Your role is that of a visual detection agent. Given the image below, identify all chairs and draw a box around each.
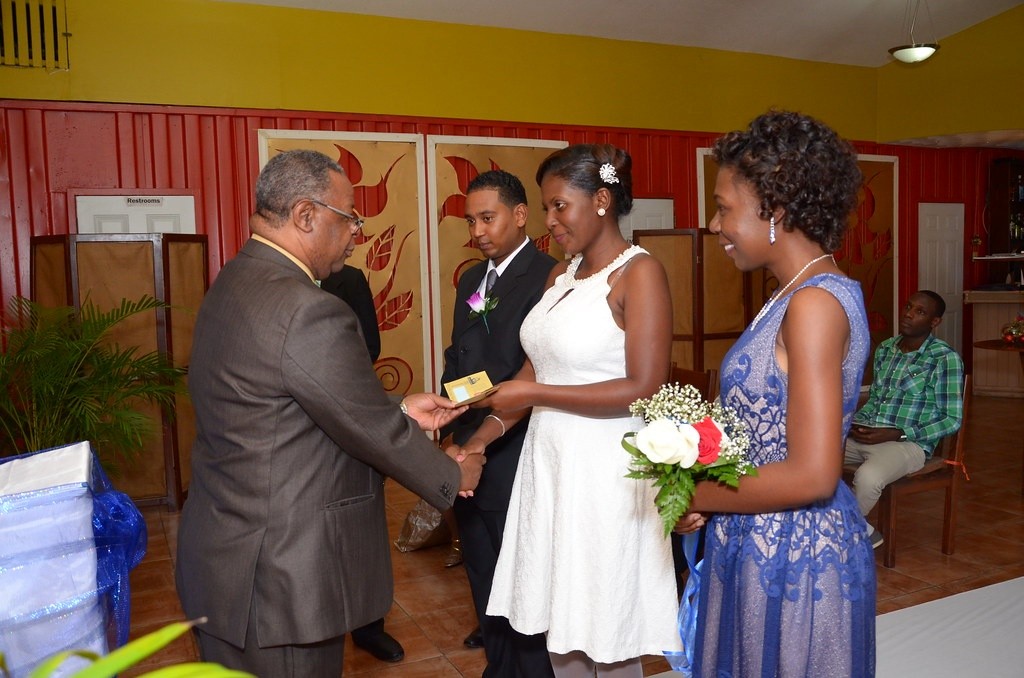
[667,365,718,403]
[840,374,972,569]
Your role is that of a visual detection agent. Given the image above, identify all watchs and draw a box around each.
[894,429,907,443]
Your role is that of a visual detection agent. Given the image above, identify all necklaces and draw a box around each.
[563,242,639,288]
[750,253,834,332]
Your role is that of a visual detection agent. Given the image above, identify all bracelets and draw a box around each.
[400,398,407,415]
[483,415,506,438]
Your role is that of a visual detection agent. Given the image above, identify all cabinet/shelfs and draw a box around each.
[1006,161,1024,289]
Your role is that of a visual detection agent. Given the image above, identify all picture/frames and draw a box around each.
[67,186,203,235]
[426,135,569,440]
[258,129,435,442]
[695,146,899,392]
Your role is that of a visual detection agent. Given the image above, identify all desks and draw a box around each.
[972,340,1024,371]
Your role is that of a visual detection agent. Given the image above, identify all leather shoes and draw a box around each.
[359,631,404,662]
[463,627,484,648]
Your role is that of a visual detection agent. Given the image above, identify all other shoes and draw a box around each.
[443,540,462,566]
[868,528,884,549]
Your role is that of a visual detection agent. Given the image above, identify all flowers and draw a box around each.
[621,381,762,541]
[466,292,500,334]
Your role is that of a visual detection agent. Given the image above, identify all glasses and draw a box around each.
[311,199,364,236]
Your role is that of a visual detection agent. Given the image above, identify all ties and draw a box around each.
[485,269,499,297]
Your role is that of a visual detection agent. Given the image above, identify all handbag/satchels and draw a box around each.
[394,500,450,553]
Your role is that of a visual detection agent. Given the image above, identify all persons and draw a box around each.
[176,149,487,678]
[839,290,966,553]
[640,111,877,678]
[451,145,688,678]
[316,169,557,678]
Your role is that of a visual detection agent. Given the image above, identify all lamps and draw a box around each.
[888,0,941,65]
